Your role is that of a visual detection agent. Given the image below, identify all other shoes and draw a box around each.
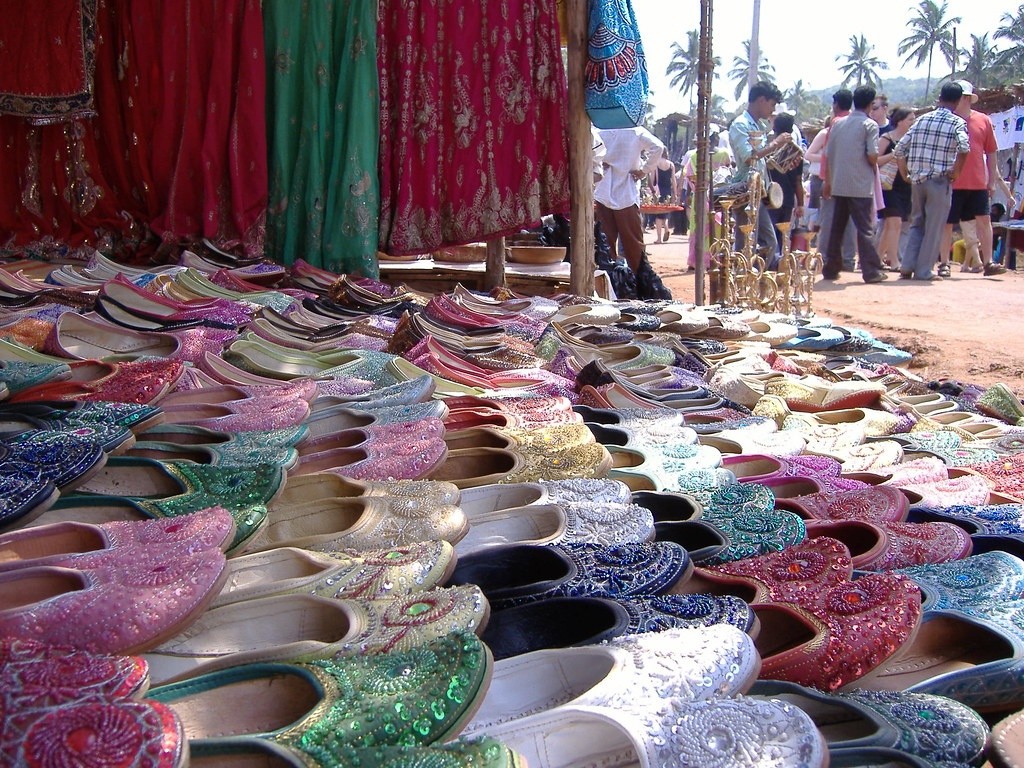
[3,232,1024,768]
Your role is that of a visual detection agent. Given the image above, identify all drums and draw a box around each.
[764,182,784,209]
[768,138,803,176]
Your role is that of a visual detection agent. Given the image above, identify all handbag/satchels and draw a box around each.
[584,1,649,130]
[645,185,659,198]
[879,156,898,190]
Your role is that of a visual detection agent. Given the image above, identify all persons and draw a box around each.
[594,126,663,276]
[640,80,1024,283]
[591,125,607,183]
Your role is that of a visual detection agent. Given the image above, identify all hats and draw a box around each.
[772,103,796,116]
[939,80,978,103]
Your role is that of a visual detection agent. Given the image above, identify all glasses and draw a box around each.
[873,105,881,110]
